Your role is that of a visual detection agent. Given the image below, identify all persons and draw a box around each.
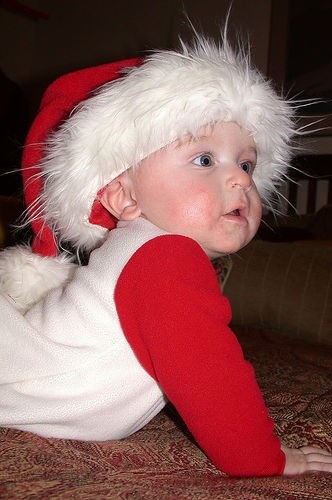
[0,36,332,475]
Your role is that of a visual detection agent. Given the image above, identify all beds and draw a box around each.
[0,155,332,500]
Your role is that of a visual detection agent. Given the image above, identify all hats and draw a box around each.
[0,48,292,310]
